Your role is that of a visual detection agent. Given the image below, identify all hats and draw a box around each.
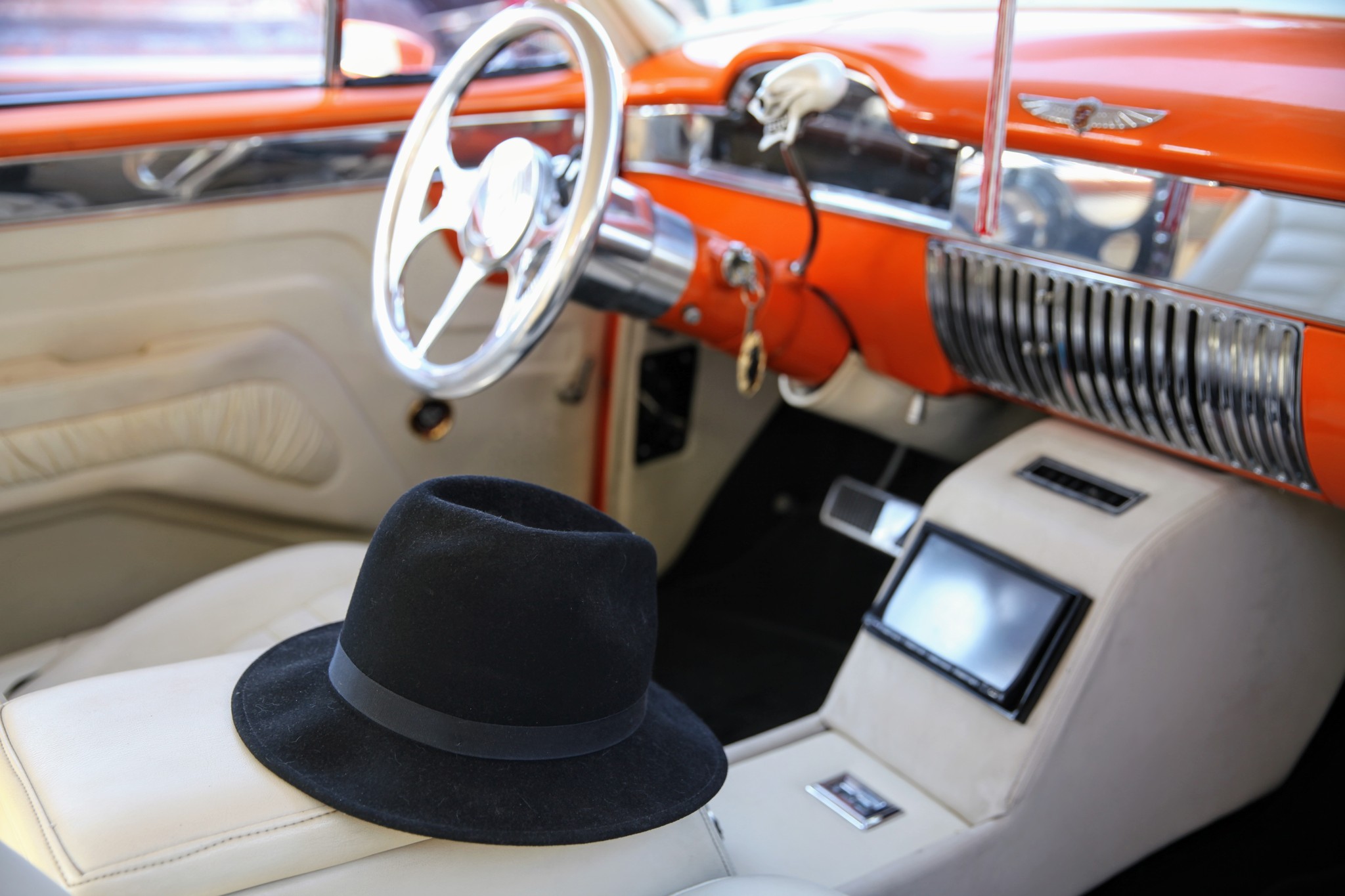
[229,475,729,847]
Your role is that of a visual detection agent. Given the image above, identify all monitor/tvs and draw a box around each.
[860,523,1074,704]
